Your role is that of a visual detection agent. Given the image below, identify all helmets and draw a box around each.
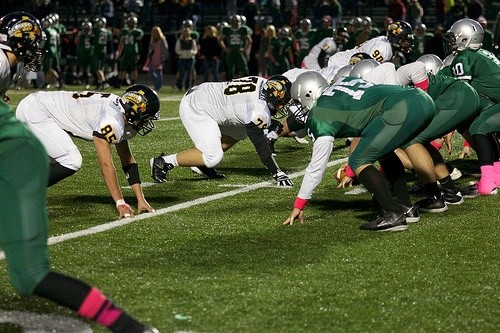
[349,60,379,79]
[40,12,373,38]
[0,11,43,53]
[117,83,161,126]
[387,20,415,46]
[416,53,443,77]
[332,64,354,83]
[291,71,328,112]
[263,75,292,107]
[447,17,484,51]
[442,53,456,67]
[332,26,349,44]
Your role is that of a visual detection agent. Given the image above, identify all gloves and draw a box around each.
[268,118,283,135]
[272,170,294,187]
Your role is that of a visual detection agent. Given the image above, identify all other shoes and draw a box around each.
[293,136,308,144]
[359,205,420,233]
[440,187,465,205]
[262,128,279,156]
[462,179,500,199]
[414,195,449,213]
[437,168,462,184]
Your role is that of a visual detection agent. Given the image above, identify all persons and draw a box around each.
[150,74,294,187]
[16,84,160,220]
[0,0,500,92]
[0,12,48,104]
[283,18,500,233]
[0,100,159,333]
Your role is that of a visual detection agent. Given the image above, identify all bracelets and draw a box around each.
[116,199,125,206]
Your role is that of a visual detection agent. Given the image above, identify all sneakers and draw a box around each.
[191,164,225,179]
[150,152,174,183]
